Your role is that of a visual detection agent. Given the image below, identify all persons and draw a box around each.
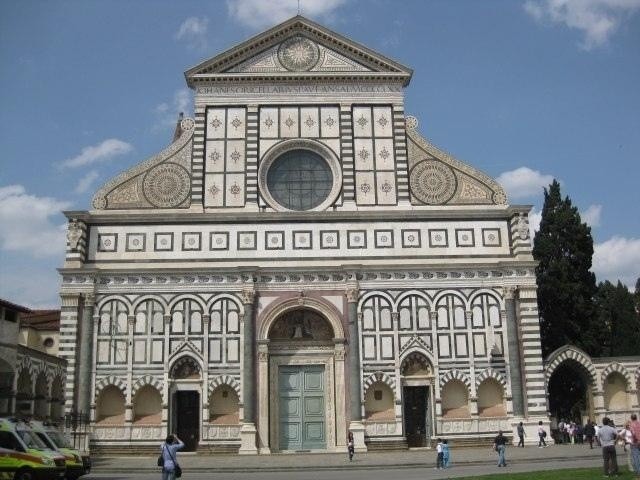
[550,417,583,445]
[346,432,354,462]
[160,433,185,480]
[584,414,640,478]
[494,431,508,467]
[517,422,527,448]
[434,438,449,470]
[537,421,547,446]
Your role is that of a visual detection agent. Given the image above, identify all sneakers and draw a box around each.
[498,464,506,467]
[601,474,618,477]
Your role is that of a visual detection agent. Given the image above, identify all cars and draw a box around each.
[0,417,91,480]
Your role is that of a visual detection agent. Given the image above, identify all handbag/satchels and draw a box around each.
[176,464,182,478]
[158,456,164,466]
